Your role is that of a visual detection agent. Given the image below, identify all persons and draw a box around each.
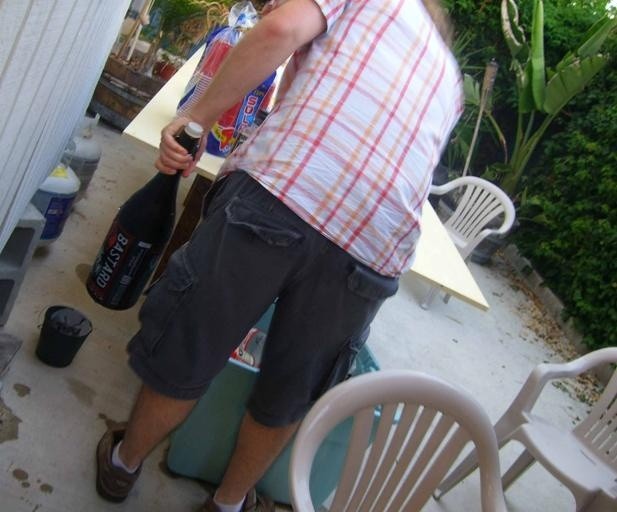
[94,1,466,512]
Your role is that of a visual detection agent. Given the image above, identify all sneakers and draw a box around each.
[94,420,144,504]
[201,488,275,512]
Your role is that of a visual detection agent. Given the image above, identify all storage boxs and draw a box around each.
[164,303,402,512]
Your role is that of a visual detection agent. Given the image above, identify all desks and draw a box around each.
[120,40,492,295]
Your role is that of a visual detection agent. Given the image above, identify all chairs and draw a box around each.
[421,175,517,310]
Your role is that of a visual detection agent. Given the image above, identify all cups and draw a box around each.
[180,38,232,120]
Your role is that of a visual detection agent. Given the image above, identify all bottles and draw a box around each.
[82,121,205,314]
[228,109,270,160]
[207,66,277,157]
